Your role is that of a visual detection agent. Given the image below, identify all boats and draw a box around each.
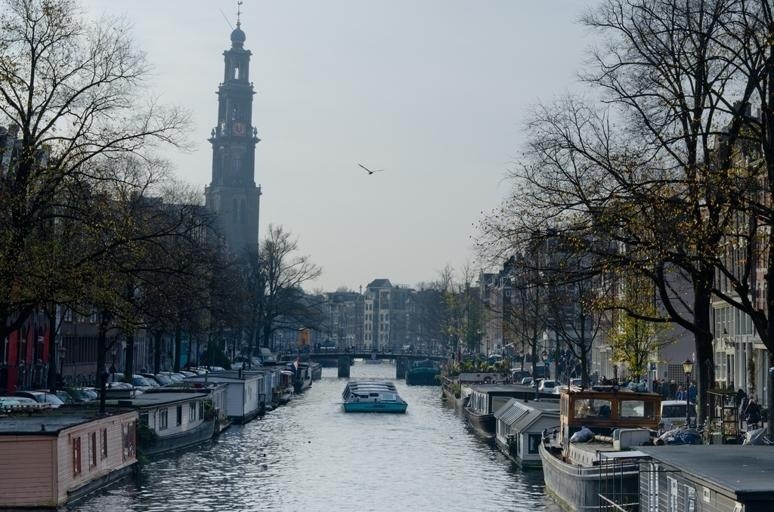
[341,381,408,413]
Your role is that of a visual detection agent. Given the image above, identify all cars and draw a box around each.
[0,345,278,413]
[510,365,698,432]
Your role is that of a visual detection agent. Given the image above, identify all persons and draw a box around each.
[601,379,762,429]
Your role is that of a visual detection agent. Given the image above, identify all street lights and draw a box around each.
[681,357,695,428]
[542,352,548,380]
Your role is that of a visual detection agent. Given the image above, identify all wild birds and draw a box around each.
[358,162,384,175]
